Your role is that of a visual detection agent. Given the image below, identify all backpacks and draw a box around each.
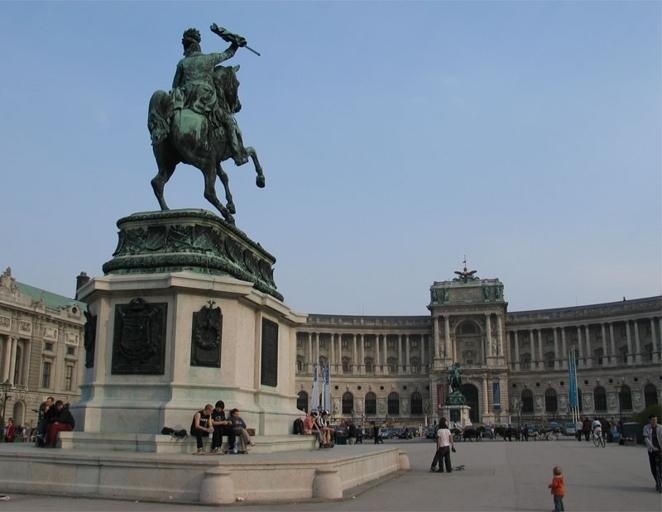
[293,416,305,435]
[161,426,188,441]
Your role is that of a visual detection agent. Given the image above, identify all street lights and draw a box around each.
[2,392,13,420]
[613,381,623,432]
[512,401,524,440]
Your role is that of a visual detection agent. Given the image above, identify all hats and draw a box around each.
[215,400,225,409]
[322,411,328,416]
[311,411,318,418]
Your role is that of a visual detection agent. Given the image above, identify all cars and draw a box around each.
[379,422,576,442]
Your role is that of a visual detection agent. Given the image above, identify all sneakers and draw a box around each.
[319,442,334,448]
[198,441,255,455]
[430,468,453,472]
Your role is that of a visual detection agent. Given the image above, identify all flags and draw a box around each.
[567,349,579,410]
[311,365,331,414]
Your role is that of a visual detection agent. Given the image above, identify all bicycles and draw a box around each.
[592,432,606,448]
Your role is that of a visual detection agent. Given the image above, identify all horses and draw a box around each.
[145,63,266,227]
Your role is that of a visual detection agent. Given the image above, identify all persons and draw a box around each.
[575,415,624,447]
[641,413,662,492]
[211,400,237,454]
[548,466,565,511]
[448,361,465,395]
[1,395,76,448]
[190,403,216,455]
[303,410,564,472]
[170,22,262,166]
[226,407,256,453]
